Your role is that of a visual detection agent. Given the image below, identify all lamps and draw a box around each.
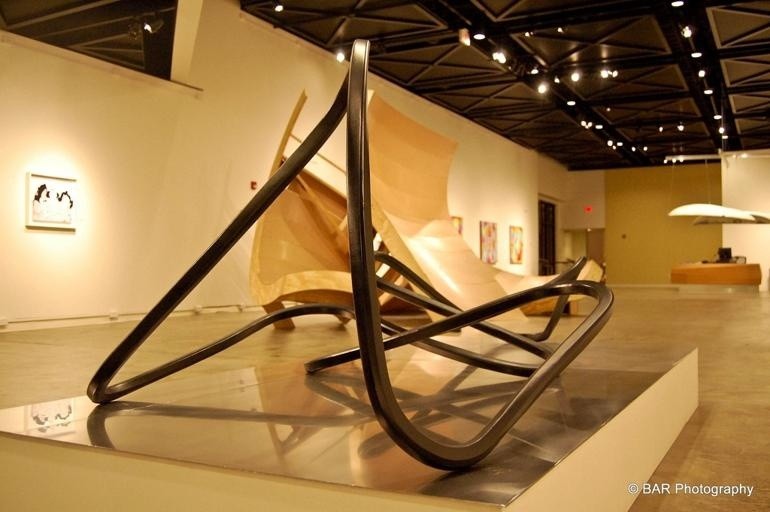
[264,0,740,163]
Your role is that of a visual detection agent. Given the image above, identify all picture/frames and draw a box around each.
[21,171,80,233]
